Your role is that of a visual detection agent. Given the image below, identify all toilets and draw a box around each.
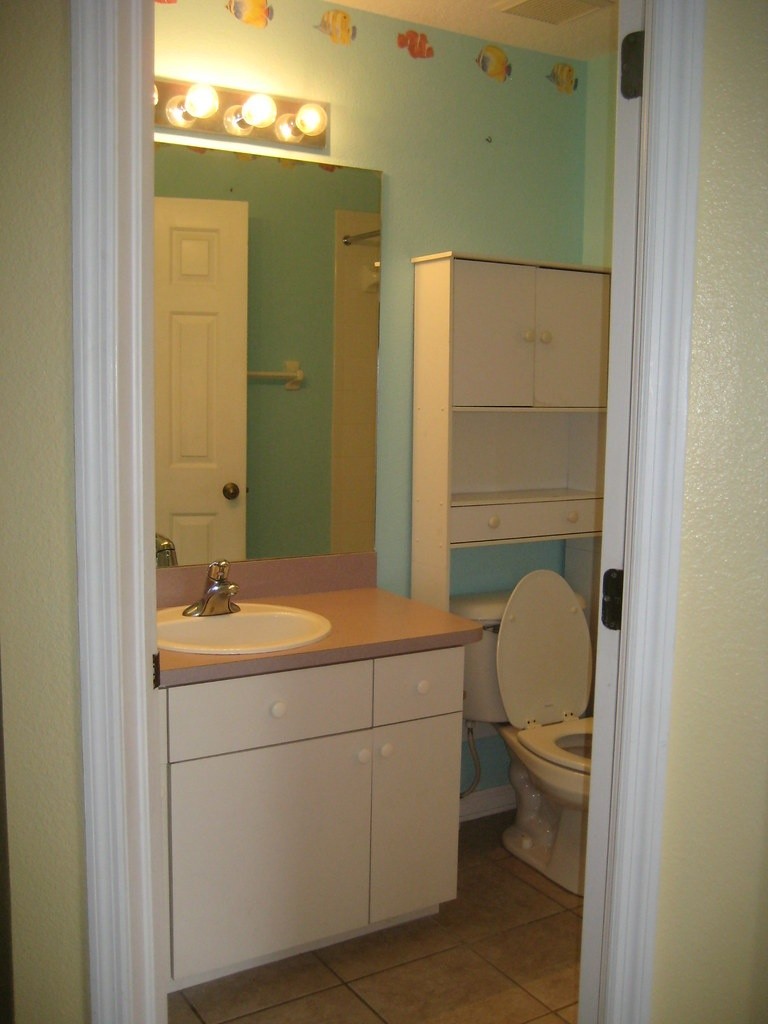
[451,568,595,894]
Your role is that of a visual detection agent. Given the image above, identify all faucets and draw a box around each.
[184,561,240,617]
[157,534,179,567]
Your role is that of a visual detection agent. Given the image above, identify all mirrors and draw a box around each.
[155,139,381,566]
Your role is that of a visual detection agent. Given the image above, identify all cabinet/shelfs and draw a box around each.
[411,252,611,796]
[162,647,466,994]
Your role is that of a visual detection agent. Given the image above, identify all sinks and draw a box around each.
[155,601,334,655]
